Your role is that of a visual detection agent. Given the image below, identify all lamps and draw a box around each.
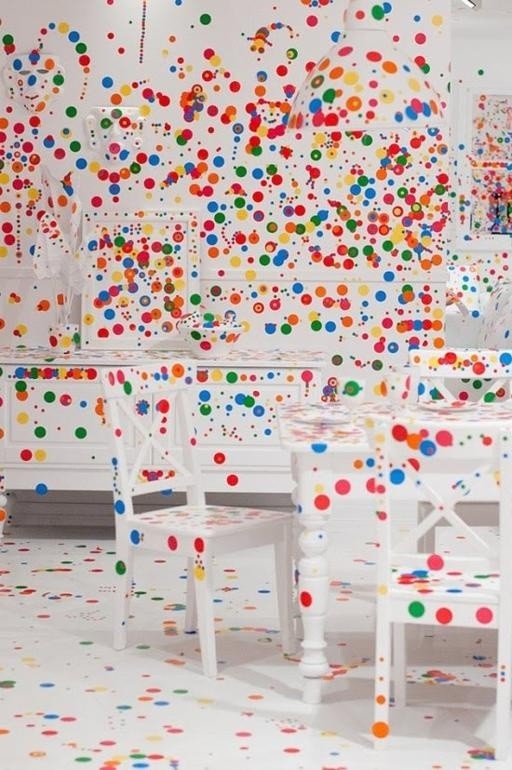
[287,0,448,136]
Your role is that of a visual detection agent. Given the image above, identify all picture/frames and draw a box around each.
[452,73,511,253]
[81,209,202,352]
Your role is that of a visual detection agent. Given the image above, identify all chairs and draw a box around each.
[101,358,297,677]
[365,415,511,762]
[408,348,512,555]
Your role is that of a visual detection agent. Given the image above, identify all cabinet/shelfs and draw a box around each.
[0,345,327,538]
